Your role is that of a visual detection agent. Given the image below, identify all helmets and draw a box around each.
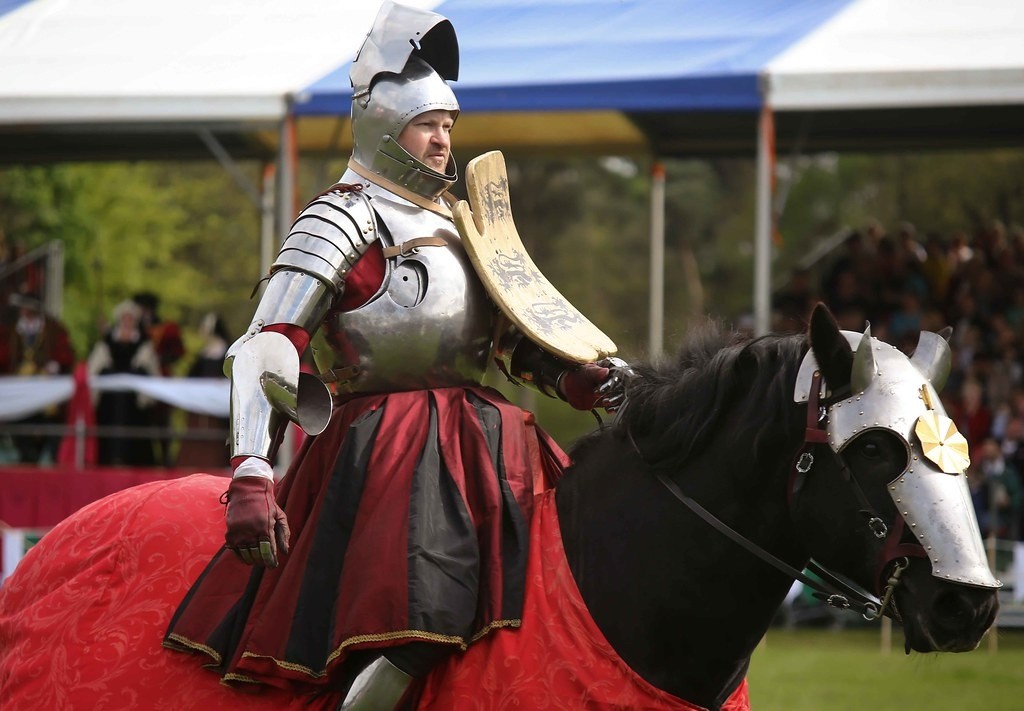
[351,0,461,201]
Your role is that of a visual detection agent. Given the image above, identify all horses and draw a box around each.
[0,299,1001,711]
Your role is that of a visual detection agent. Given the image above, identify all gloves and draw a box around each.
[220,476,291,567]
[563,356,634,415]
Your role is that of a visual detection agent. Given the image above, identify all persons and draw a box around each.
[753,215,1024,567]
[163,4,639,711]
[0,274,235,469]
